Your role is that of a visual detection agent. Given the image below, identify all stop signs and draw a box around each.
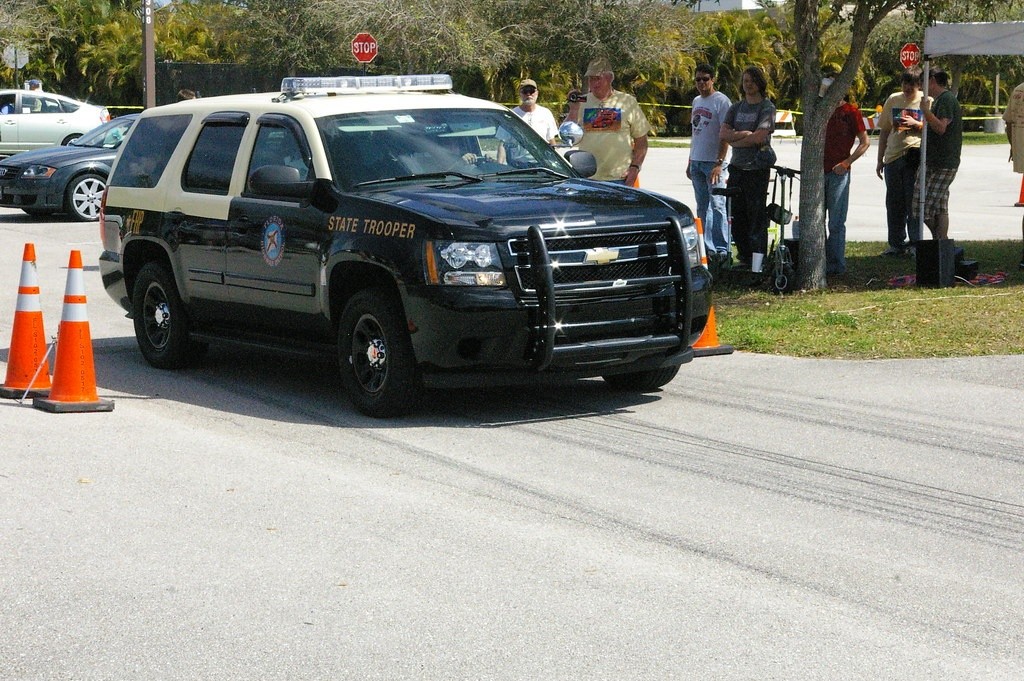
[900,43,921,69]
[351,34,378,63]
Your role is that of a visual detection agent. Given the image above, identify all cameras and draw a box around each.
[570,92,588,103]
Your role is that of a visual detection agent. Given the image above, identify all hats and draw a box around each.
[519,79,537,90]
[584,58,611,76]
[29,80,39,86]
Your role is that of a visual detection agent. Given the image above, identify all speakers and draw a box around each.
[916,238,956,290]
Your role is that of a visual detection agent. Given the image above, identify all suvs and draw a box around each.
[98,73,718,420]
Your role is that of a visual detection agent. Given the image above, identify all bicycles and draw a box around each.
[707,165,803,292]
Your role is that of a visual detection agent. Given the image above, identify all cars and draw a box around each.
[0,113,142,222]
[0,88,111,158]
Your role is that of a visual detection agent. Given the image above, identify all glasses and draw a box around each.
[521,88,536,94]
[695,77,711,82]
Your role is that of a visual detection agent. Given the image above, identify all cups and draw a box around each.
[752,253,763,272]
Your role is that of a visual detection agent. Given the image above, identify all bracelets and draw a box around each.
[840,162,849,169]
[717,159,723,163]
[630,165,639,169]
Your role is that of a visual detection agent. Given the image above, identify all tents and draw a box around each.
[919,17,1024,238]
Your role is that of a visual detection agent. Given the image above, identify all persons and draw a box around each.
[1002,82,1024,266]
[824,95,869,273]
[0,95,15,115]
[563,57,651,187]
[177,89,197,103]
[722,67,775,268]
[495,79,557,166]
[876,66,934,256]
[433,131,478,172]
[912,70,962,240]
[685,64,733,253]
[30,80,43,113]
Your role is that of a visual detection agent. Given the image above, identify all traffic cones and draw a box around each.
[1014,174,1024,208]
[686,215,736,356]
[0,242,53,400]
[33,249,117,413]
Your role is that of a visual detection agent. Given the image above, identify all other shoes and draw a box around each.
[883,247,901,255]
